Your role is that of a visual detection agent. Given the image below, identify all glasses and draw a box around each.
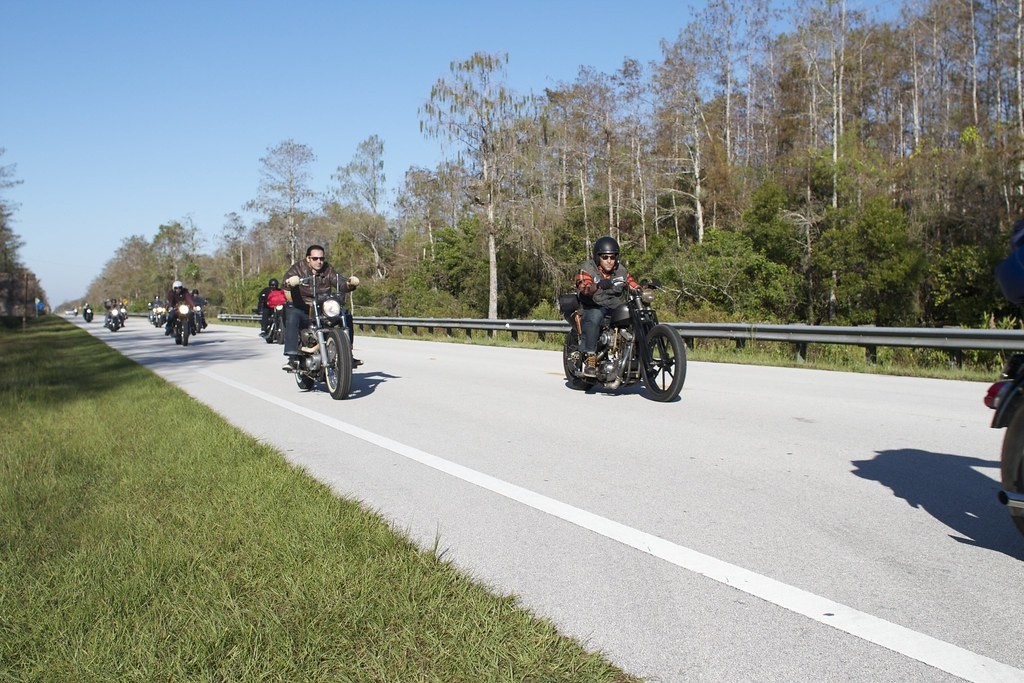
[174,287,182,289]
[270,284,277,287]
[598,254,616,260]
[310,256,326,261]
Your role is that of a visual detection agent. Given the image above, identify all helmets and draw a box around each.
[592,237,621,269]
[269,278,279,289]
[192,289,199,295]
[172,280,184,292]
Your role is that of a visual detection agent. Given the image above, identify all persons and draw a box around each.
[83,303,93,320]
[576,237,643,377]
[259,279,281,335]
[281,245,364,371]
[104,299,126,327]
[149,281,208,335]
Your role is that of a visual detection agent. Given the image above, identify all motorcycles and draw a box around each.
[166,300,196,347]
[289,273,363,399]
[84,308,94,323]
[558,278,688,404]
[105,306,128,333]
[148,303,167,328]
[251,290,293,345]
[984,353,1023,537]
[192,300,209,334]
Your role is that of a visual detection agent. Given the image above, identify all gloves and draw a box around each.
[346,276,361,289]
[285,274,300,286]
[597,278,614,290]
[641,277,662,289]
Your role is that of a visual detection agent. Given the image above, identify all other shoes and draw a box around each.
[352,357,364,368]
[258,332,266,337]
[165,329,171,336]
[283,357,297,372]
[190,330,197,335]
[583,353,597,378]
